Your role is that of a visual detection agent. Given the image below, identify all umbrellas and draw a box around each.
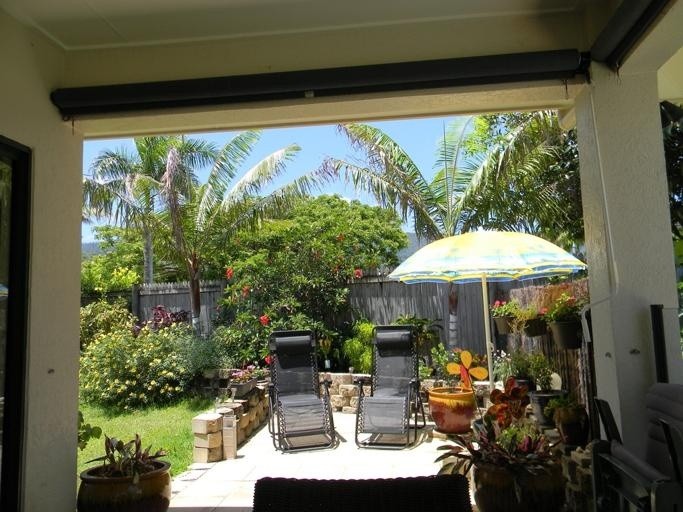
[387,231,588,395]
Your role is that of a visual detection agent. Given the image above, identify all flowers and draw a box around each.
[435,418,556,478]
[231,365,255,384]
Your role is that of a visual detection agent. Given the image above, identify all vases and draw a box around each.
[467,461,568,512]
[229,376,258,397]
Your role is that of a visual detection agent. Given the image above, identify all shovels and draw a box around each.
[592,440,683,512]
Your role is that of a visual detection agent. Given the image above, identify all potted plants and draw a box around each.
[491,293,589,445]
[77,433,172,512]
[428,342,477,434]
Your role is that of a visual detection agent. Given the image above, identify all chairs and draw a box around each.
[252,473,473,511]
[268,330,336,453]
[355,325,426,449]
